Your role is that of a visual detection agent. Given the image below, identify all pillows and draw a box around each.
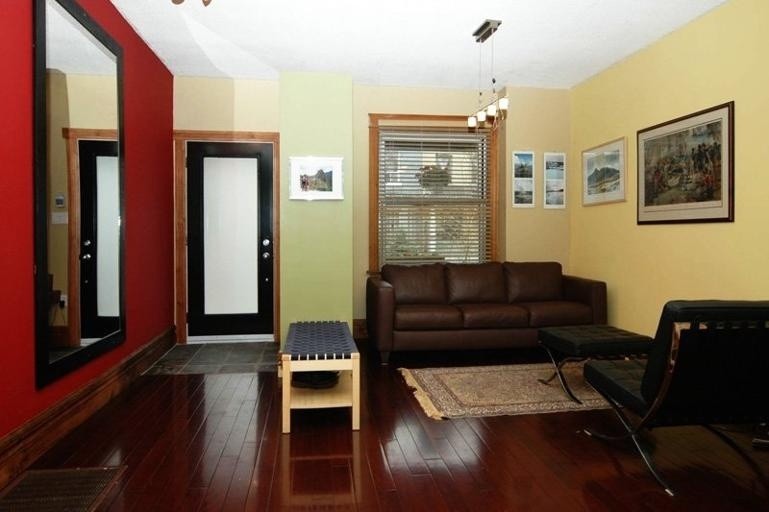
[382,262,447,305]
[445,262,506,305]
[502,261,561,305]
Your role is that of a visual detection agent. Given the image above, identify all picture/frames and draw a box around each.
[512,151,534,209]
[544,153,566,209]
[581,137,628,207]
[638,101,735,225]
[288,155,346,201]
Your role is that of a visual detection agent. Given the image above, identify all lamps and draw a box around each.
[467,19,509,132]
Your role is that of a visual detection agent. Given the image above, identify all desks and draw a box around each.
[278,321,361,434]
[281,434,363,506]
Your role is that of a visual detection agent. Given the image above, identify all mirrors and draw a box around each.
[34,0,127,391]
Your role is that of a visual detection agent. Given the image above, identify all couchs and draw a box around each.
[366,262,607,367]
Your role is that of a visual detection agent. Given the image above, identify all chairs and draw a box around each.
[583,300,768,496]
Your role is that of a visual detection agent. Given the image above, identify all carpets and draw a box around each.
[0,465,128,511]
[397,361,624,421]
